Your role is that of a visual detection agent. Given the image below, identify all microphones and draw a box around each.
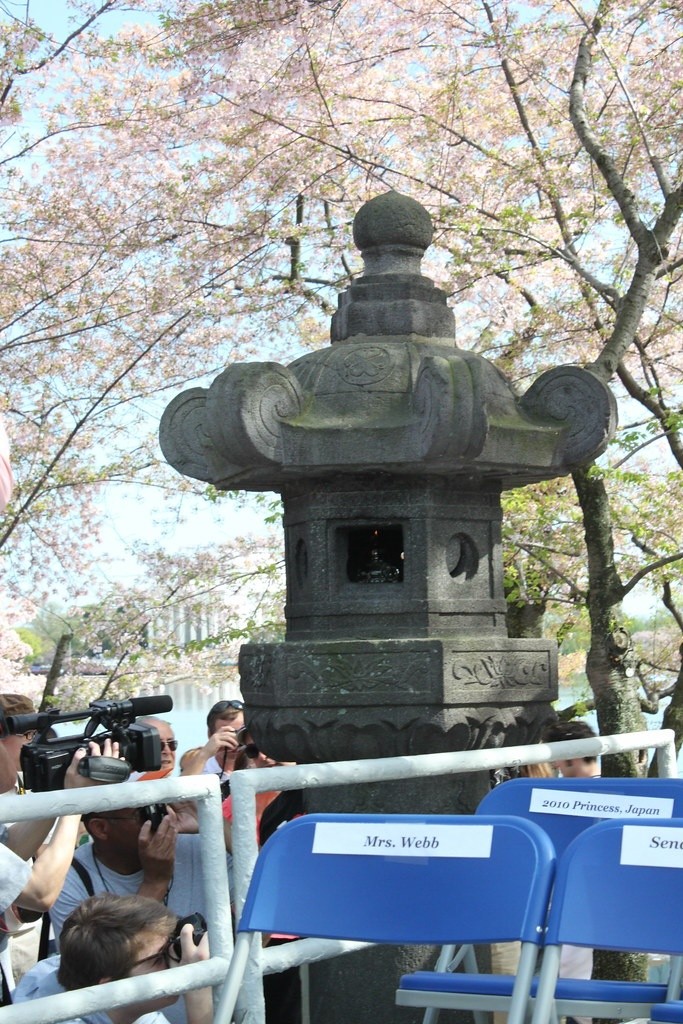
[130,695,174,717]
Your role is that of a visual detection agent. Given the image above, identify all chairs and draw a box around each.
[394,777,683,1024]
[529,817,683,1024]
[210,811,555,1024]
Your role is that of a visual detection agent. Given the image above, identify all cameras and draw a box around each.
[238,725,246,747]
[173,912,207,959]
[18,723,162,791]
[141,802,170,830]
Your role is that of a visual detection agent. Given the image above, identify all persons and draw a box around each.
[48,804,273,1023]
[0,693,59,865]
[488,720,602,1024]
[131,699,297,849]
[14,891,217,1024]
[221,721,304,1024]
[1,736,126,1000]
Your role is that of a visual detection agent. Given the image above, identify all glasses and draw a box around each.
[244,744,258,760]
[159,740,179,752]
[127,939,174,972]
[213,701,244,714]
[96,810,144,824]
